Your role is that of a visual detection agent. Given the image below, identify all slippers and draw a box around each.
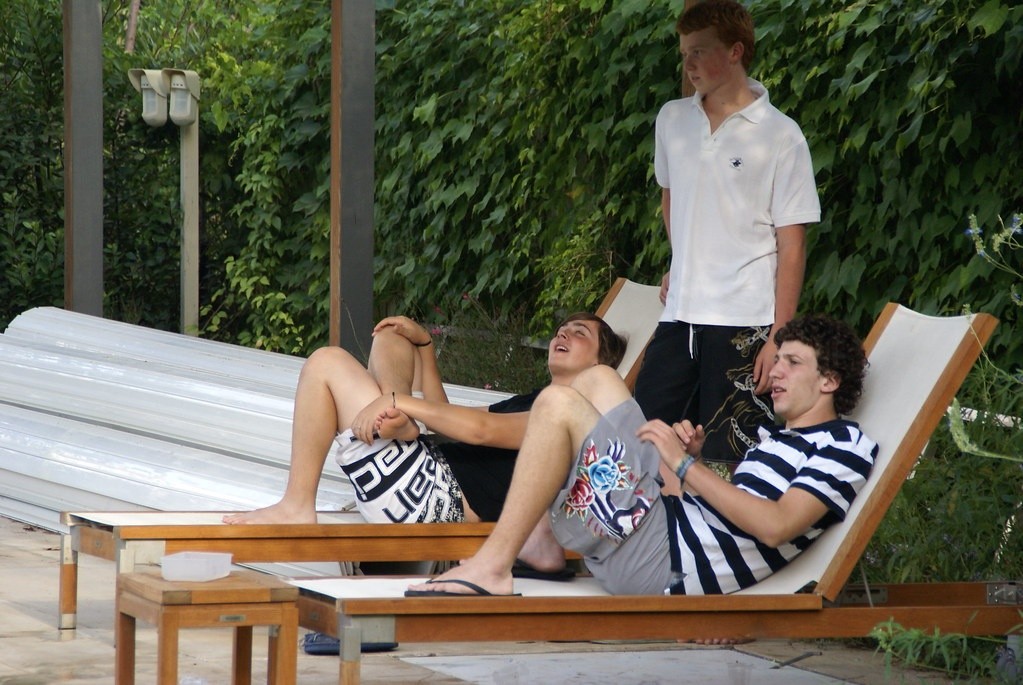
[404,577,522,597]
[455,558,576,581]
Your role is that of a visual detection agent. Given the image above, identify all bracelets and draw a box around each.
[416,340,432,347]
[675,454,695,478]
[392,392,395,408]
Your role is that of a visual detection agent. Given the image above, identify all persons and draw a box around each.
[406,312,878,598]
[630,1,823,648]
[221,314,629,530]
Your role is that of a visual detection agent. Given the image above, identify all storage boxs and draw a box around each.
[159,550,233,582]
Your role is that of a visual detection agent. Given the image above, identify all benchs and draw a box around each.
[57,276,664,632]
[283,301,1023,685]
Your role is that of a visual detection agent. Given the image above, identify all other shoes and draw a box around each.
[304,632,399,654]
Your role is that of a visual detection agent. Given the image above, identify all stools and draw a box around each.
[115,566,299,685]
